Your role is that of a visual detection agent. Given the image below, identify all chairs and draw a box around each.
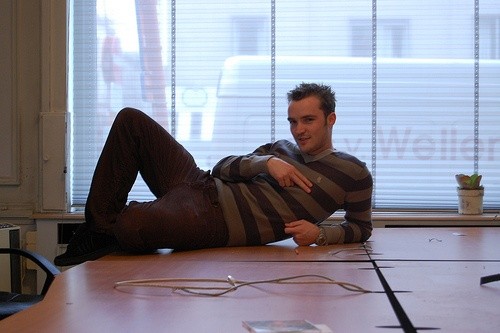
[0,247,61,316]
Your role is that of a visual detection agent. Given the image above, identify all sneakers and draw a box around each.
[54,224,114,266]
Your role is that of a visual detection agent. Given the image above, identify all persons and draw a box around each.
[57,82,374,267]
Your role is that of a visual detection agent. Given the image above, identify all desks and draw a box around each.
[0,228,500,333]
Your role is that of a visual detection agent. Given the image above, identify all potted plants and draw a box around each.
[455,174,484,214]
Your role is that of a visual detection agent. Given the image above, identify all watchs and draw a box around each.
[316,226,327,245]
